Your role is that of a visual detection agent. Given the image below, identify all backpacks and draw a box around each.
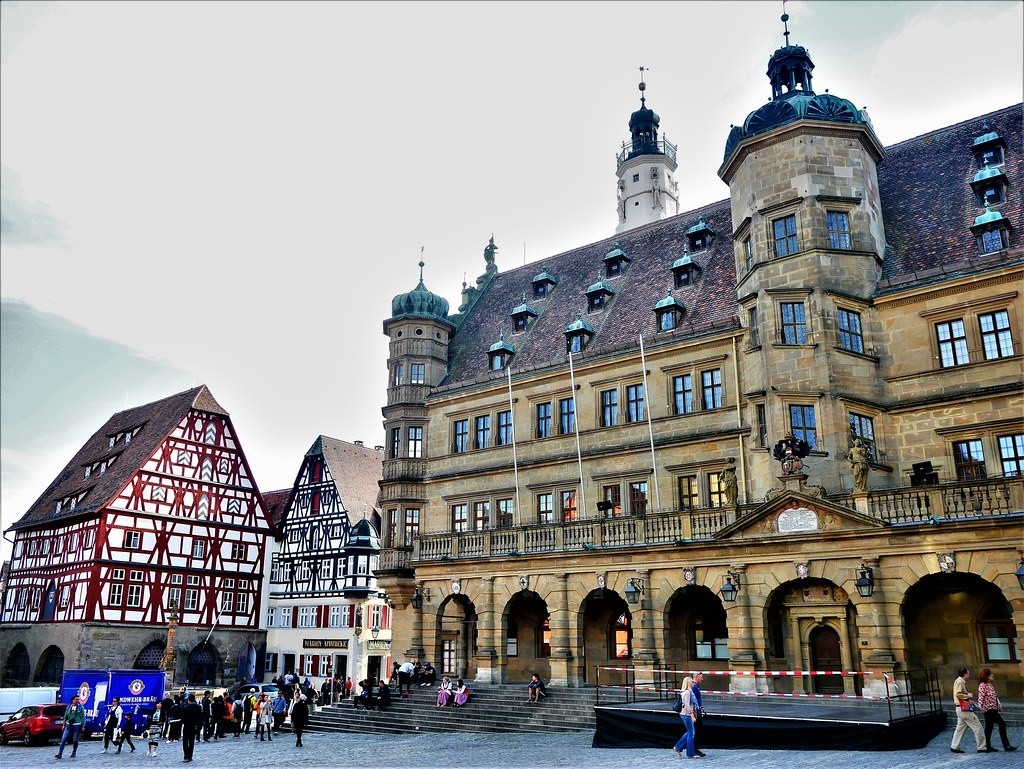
[233,702,243,719]
[244,695,254,712]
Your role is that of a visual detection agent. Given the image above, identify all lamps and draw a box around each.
[1014,557,1024,590]
[624,578,645,604]
[371,626,380,638]
[855,563,875,597]
[410,586,430,609]
[720,570,741,602]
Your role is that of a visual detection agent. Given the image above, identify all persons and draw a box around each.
[674,672,706,759]
[528,674,546,703]
[950,667,989,753]
[162,669,352,747]
[137,701,165,756]
[452,679,469,707]
[389,661,401,688]
[181,694,203,763]
[100,697,123,754]
[846,439,868,487]
[398,660,414,700]
[353,679,373,709]
[376,680,390,706]
[114,712,136,753]
[55,695,86,759]
[718,457,738,504]
[437,677,453,707]
[410,662,436,687]
[976,669,1017,752]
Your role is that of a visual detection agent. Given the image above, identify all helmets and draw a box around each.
[424,661,431,667]
[249,687,255,692]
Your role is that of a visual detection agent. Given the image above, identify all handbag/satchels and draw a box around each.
[113,727,122,742]
[257,717,261,723]
[672,694,683,713]
[959,698,977,712]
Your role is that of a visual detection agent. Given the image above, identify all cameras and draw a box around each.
[68,718,75,725]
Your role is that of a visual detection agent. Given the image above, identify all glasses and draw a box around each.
[112,700,117,702]
[74,698,79,700]
[696,672,702,677]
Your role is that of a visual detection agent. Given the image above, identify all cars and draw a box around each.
[230,684,284,702]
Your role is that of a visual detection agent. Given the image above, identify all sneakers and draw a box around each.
[100,747,108,754]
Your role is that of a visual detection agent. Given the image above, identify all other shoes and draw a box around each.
[1004,745,1018,752]
[950,747,965,754]
[152,751,155,756]
[146,751,150,756]
[673,746,683,759]
[115,749,121,755]
[695,750,706,757]
[165,732,278,744]
[70,750,76,758]
[130,748,135,752]
[978,749,989,753]
[988,746,999,753]
[55,753,62,759]
[688,755,699,758]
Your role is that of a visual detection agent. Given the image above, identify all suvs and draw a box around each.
[1,704,69,745]
